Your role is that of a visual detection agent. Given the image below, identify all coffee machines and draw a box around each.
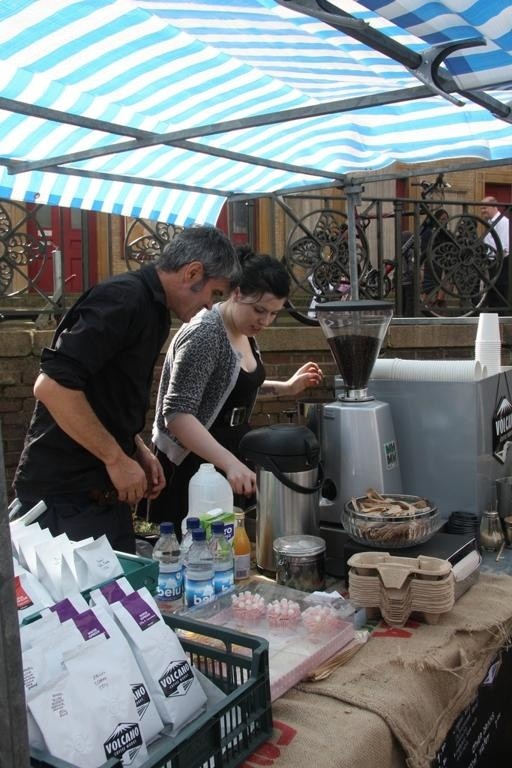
[315,298,404,526]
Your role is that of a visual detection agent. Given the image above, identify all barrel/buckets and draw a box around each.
[181,463,235,542]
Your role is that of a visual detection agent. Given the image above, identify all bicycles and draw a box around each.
[277,172,492,327]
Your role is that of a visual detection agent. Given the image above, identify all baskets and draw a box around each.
[29,610,275,767]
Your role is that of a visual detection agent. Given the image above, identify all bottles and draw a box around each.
[502,517,512,548]
[480,505,504,552]
[183,526,216,610]
[231,508,251,580]
[208,519,236,609]
[186,462,234,516]
[179,516,200,557]
[152,520,184,613]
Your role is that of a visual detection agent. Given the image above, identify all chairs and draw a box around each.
[306,271,348,321]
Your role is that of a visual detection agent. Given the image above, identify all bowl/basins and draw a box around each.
[341,491,440,546]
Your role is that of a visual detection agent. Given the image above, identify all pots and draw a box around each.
[296,396,336,433]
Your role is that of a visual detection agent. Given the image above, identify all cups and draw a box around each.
[474,312,501,374]
[369,357,488,381]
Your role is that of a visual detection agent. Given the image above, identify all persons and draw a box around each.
[478,196,509,312]
[137,244,325,540]
[12,224,242,554]
[330,224,362,278]
[419,211,455,309]
